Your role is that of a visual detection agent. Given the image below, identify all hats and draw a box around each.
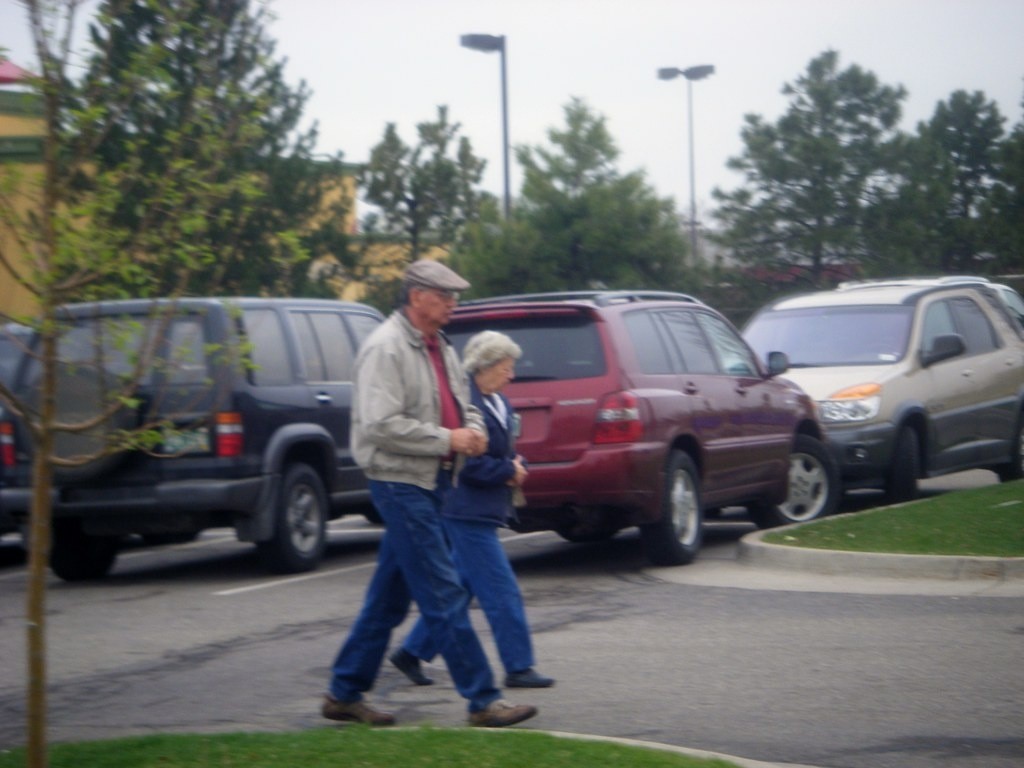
[402,258,472,289]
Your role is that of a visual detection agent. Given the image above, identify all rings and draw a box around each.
[466,449,472,455]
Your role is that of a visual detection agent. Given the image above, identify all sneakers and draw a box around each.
[321,695,394,725]
[469,698,537,728]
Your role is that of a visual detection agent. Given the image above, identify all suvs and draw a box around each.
[439,286,840,566]
[723,275,1024,505]
[0,294,393,587]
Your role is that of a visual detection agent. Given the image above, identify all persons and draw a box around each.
[389,330,556,689]
[320,262,540,727]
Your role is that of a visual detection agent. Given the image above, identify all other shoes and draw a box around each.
[505,668,555,687]
[389,648,434,686]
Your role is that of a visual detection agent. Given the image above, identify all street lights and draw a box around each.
[459,33,515,226]
[656,64,715,297]
